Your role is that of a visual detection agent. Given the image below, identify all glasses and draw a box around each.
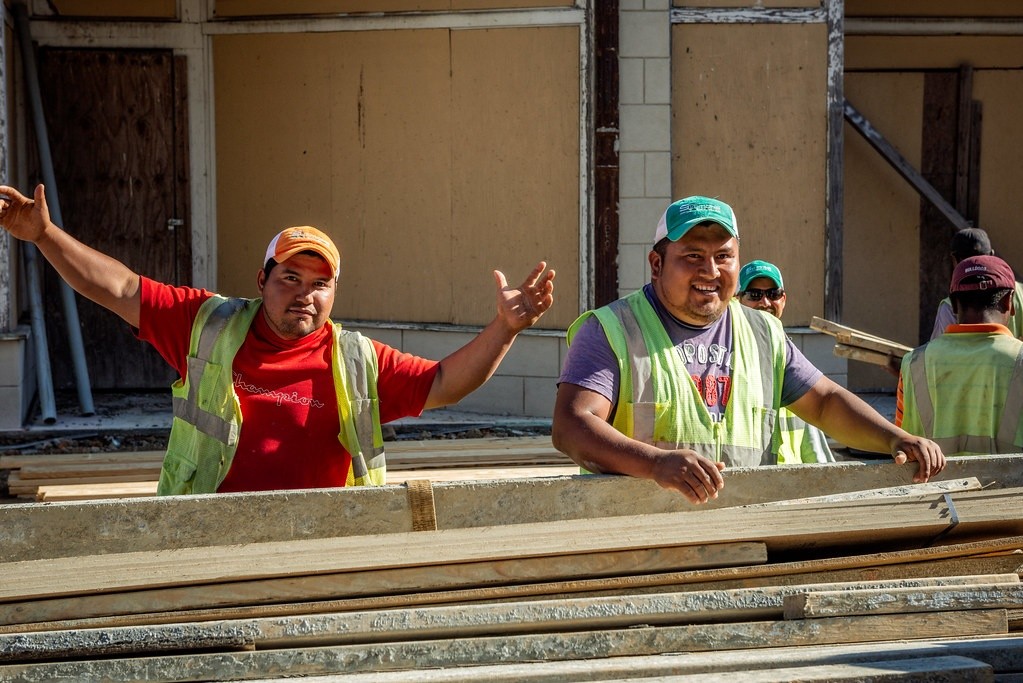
[745,288,785,301]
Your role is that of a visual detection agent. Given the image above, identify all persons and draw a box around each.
[895,253,1022,458]
[928,228,1022,343]
[1,181,555,501]
[551,196,948,506]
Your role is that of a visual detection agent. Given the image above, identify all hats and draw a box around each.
[263,225,341,284]
[736,260,784,296]
[952,228,991,255]
[950,255,1016,316]
[654,195,740,246]
